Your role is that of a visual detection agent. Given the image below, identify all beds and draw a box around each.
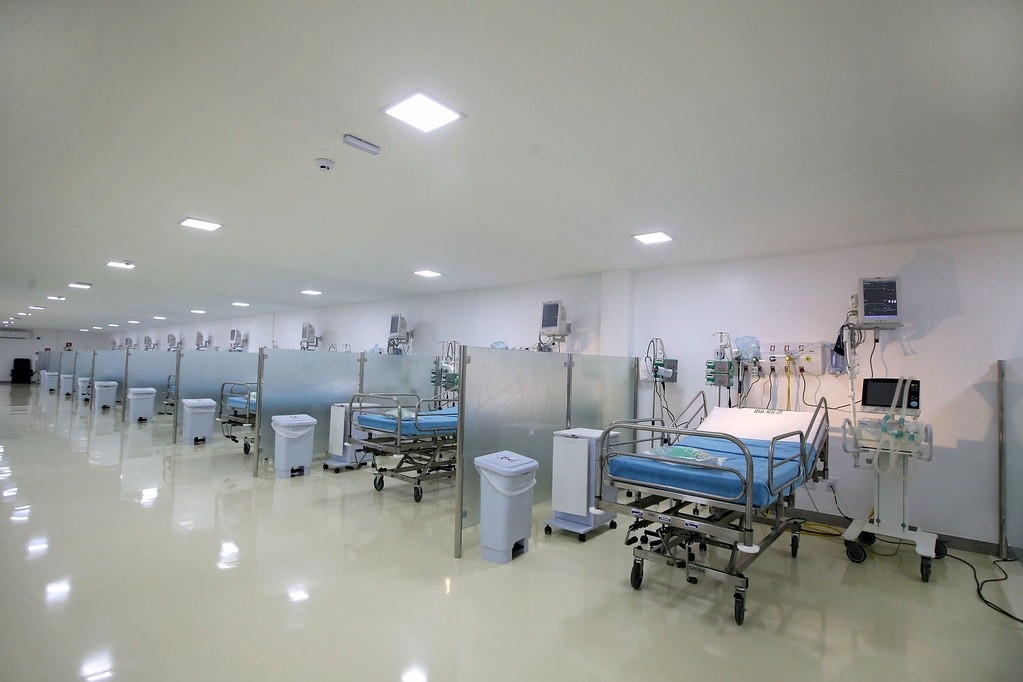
[345,391,458,504]
[216,381,257,453]
[598,390,829,626]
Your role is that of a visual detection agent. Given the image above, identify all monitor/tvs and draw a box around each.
[196,332,203,346]
[229,330,241,345]
[167,333,176,346]
[145,336,151,347]
[390,313,408,341]
[862,378,921,418]
[111,340,116,349]
[301,323,315,343]
[126,338,133,346]
[540,301,568,337]
[858,277,900,325]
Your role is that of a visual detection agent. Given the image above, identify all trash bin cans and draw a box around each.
[271,414,318,480]
[77,377,90,400]
[181,398,217,446]
[45,372,58,396]
[93,380,118,410]
[59,374,73,398]
[474,450,540,564]
[128,387,157,425]
